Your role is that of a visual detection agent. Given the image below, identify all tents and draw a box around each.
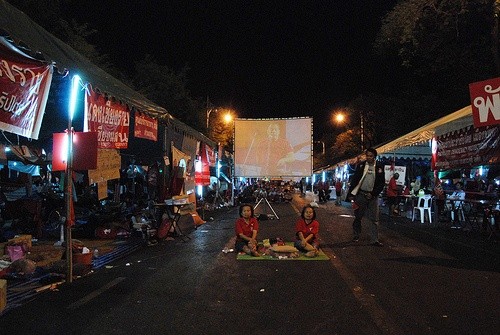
[0,2,234,282]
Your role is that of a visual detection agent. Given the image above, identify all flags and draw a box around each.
[431,132,437,169]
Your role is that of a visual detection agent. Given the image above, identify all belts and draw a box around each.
[360,188,372,193]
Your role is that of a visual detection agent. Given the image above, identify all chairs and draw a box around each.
[411,194,432,222]
[485,205,500,240]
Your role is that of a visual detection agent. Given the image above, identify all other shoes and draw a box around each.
[368,239,384,247]
[353,239,361,246]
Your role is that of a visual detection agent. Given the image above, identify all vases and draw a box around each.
[72,253,92,265]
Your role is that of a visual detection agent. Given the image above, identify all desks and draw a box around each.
[154,202,194,239]
[448,198,488,233]
[445,190,492,198]
[400,195,421,217]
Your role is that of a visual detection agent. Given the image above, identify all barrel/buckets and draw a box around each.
[77,252,92,264]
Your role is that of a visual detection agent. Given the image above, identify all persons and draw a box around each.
[318,180,327,204]
[335,178,342,201]
[235,203,260,257]
[402,176,417,211]
[299,178,308,197]
[462,169,471,192]
[453,170,463,184]
[446,181,465,228]
[386,173,400,218]
[240,180,295,204]
[436,174,454,213]
[294,205,319,257]
[324,181,332,201]
[344,147,385,246]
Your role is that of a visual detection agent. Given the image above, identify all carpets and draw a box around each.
[236,242,330,261]
